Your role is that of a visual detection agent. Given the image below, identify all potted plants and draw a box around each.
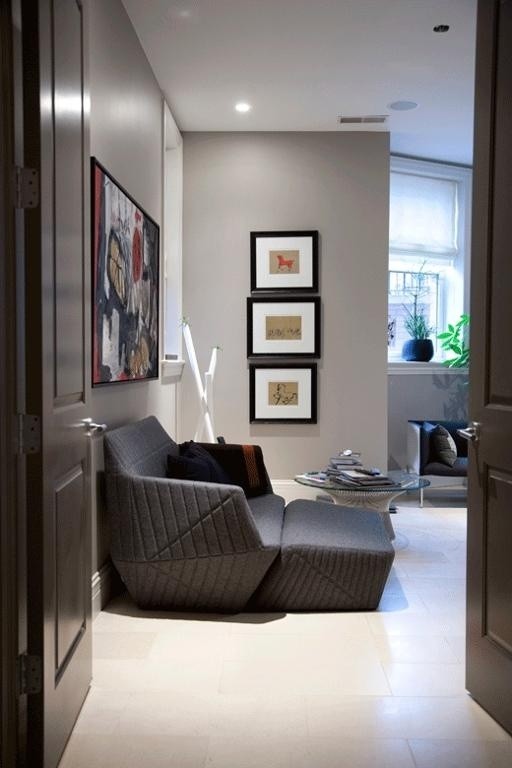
[399,260,438,362]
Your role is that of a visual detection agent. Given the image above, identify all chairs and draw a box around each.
[103,414,395,614]
[402,415,472,507]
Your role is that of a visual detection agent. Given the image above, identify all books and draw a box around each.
[297,454,396,489]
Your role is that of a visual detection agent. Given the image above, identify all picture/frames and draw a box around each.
[245,228,322,423]
[91,154,161,386]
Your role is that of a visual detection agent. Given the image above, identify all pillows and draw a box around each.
[166,437,273,500]
[421,421,459,468]
